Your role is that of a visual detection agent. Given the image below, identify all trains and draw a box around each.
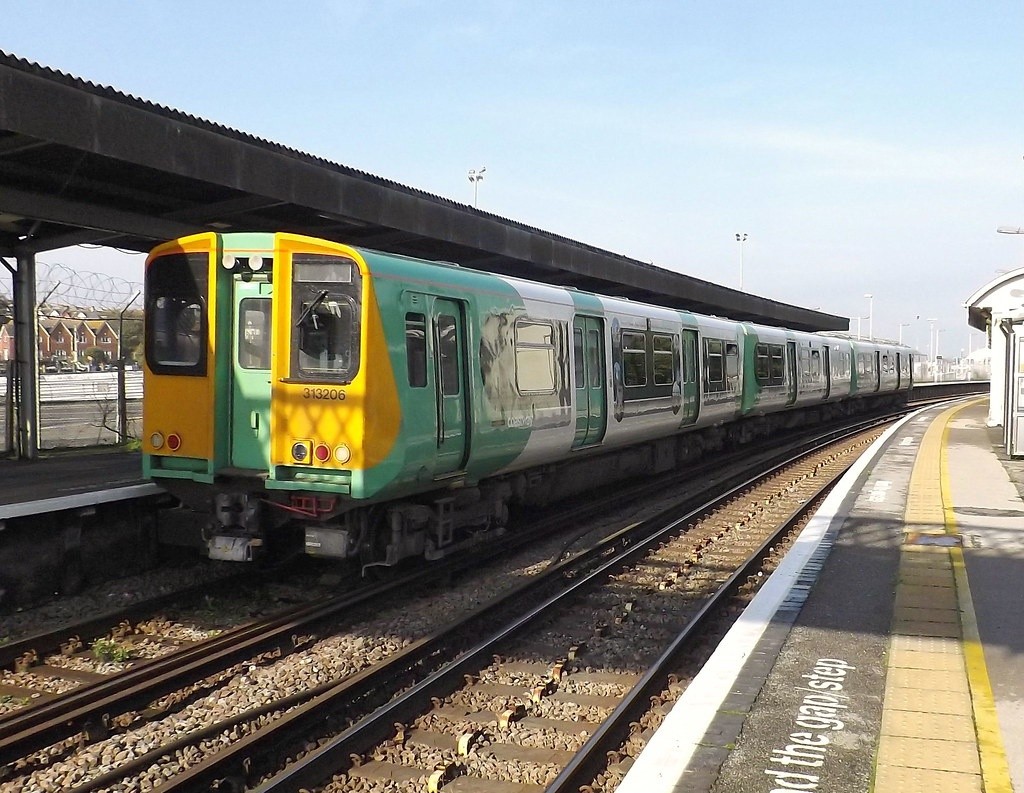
[124,226,916,591]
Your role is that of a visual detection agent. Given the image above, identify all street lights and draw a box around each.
[467,166,486,207]
[857,315,870,340]
[863,294,874,340]
[735,232,749,292]
[899,323,910,345]
